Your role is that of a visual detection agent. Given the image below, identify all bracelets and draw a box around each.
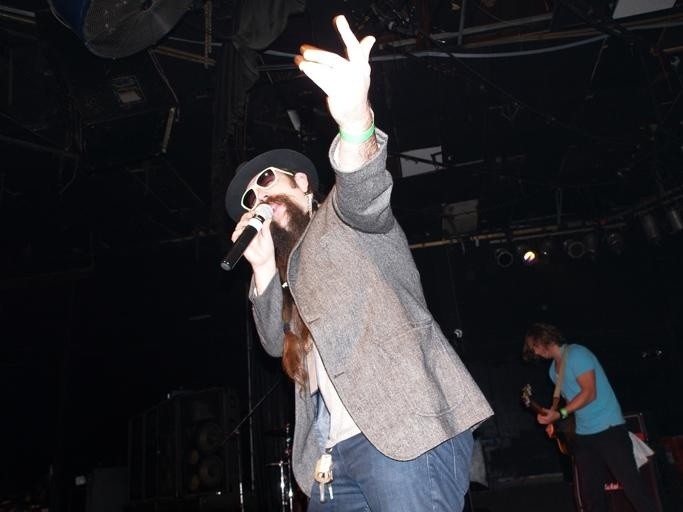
[339,108,374,145]
[560,407,569,419]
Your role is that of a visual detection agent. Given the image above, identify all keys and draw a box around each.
[319,454,335,499]
[314,453,325,502]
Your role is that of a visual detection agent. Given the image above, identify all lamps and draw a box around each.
[494,206,683,268]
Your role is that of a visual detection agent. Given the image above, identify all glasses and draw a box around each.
[238,166,294,213]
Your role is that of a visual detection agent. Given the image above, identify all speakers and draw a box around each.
[574,413,667,512]
[128,388,240,504]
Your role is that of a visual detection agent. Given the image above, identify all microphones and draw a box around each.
[220,204,273,271]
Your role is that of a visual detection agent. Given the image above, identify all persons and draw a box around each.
[224,15,497,510]
[519,321,643,510]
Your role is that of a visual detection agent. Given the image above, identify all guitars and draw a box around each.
[521,382,575,455]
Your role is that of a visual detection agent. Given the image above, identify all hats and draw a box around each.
[223,148,320,226]
